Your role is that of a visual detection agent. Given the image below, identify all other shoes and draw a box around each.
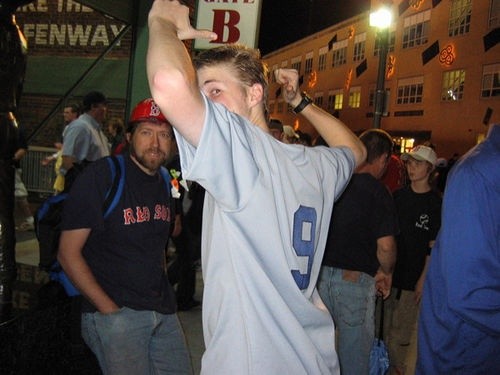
[182,298,202,311]
[16,221,36,232]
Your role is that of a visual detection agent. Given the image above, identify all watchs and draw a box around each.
[292,91,315,114]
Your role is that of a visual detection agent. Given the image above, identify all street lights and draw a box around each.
[368,6,394,131]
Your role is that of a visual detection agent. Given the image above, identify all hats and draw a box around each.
[82,91,112,106]
[129,98,167,122]
[408,144,437,166]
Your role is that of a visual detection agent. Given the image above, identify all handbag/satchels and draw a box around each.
[368,288,390,375]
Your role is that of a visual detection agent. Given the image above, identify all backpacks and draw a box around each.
[34,154,172,297]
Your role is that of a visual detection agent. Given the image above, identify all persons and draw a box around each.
[316,130,442,375]
[42,92,127,197]
[168,164,206,310]
[268,119,329,148]
[417,126,500,375]
[147,0,367,375]
[55,96,195,375]
[9,115,34,231]
